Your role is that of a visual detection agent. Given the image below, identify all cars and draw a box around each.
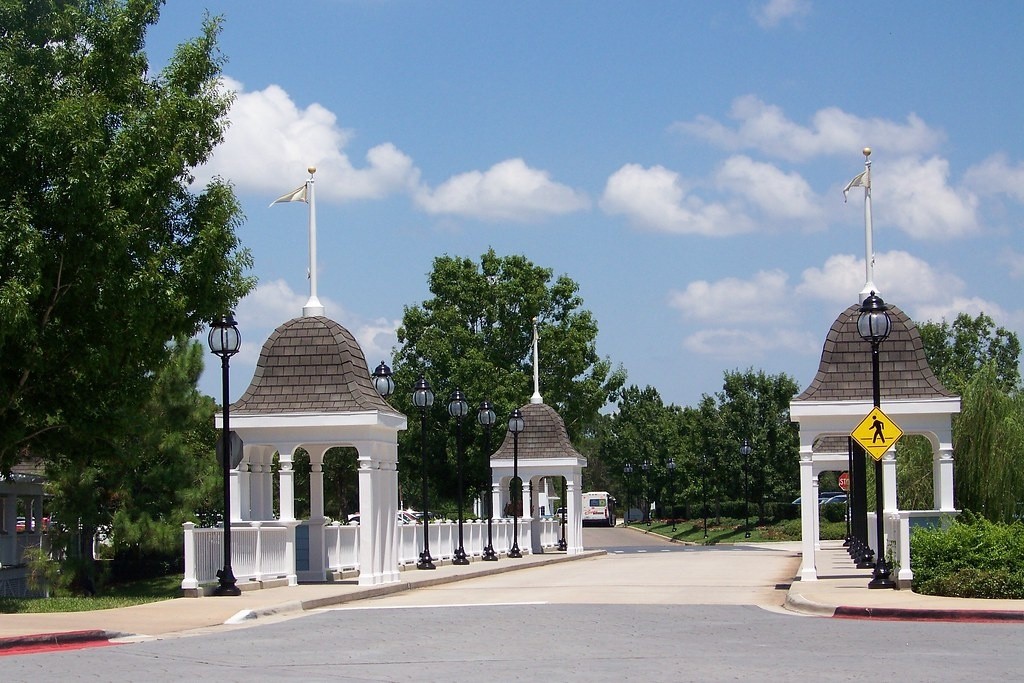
[554,507,567,522]
[793,491,851,509]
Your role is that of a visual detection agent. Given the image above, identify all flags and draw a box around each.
[843,170,871,203]
[270,184,308,209]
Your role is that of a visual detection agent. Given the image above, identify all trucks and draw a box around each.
[582,492,616,526]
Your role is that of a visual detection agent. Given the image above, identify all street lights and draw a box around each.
[642,458,651,525]
[699,453,710,539]
[447,386,470,565]
[413,375,437,569]
[855,291,896,589]
[207,308,241,596]
[506,406,524,558]
[739,437,752,538]
[622,462,633,524]
[476,401,498,561]
[667,457,677,532]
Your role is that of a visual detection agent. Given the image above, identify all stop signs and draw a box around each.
[839,470,851,492]
